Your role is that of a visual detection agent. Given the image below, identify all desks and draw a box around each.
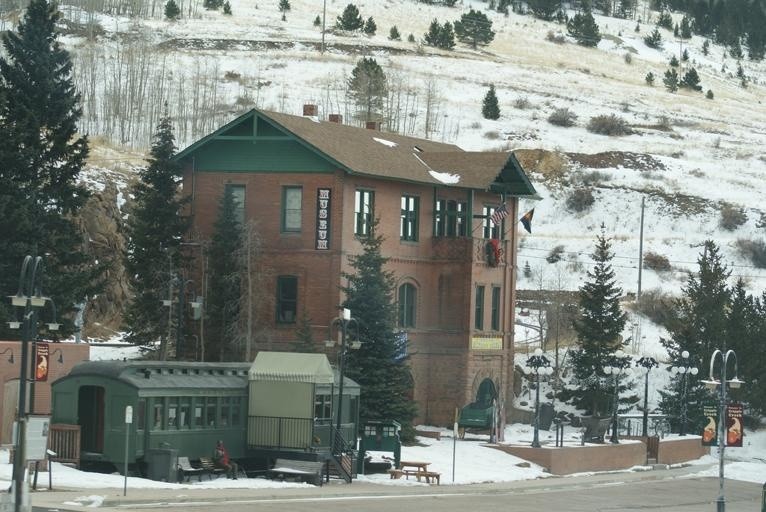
[398,461,432,483]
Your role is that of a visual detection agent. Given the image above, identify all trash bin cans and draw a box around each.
[148,447,178,482]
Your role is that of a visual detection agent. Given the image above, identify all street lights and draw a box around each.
[701,350,746,512]
[159,276,203,361]
[604,350,633,444]
[6,255,62,512]
[634,349,660,436]
[324,307,369,456]
[670,350,698,436]
[524,348,553,448]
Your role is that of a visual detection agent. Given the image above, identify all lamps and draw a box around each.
[49,348,63,364]
[0,348,14,364]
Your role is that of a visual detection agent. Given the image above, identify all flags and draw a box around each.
[490,205,509,225]
[520,208,534,235]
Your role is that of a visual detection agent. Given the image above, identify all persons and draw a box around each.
[214,440,238,480]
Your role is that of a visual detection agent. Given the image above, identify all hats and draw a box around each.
[217,440,223,445]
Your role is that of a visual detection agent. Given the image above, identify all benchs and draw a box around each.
[200,457,247,480]
[268,458,329,487]
[388,470,442,486]
[177,456,204,484]
